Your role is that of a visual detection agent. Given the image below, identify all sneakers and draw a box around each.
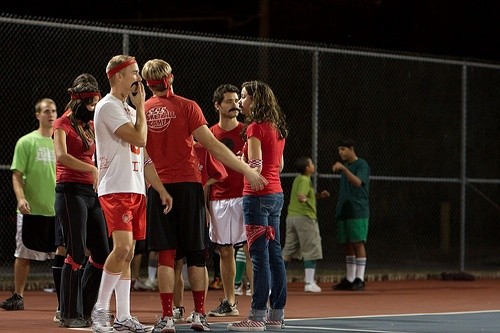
[54,310,92,328]
[227,316,284,332]
[0,293,24,310]
[332,277,365,290]
[186,312,194,322]
[235,284,251,296]
[172,306,185,323]
[208,300,238,316]
[305,282,321,291]
[183,280,192,290]
[113,316,152,333]
[91,307,118,333]
[150,317,175,333]
[187,312,211,331]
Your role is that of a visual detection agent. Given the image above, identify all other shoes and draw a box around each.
[212,279,220,289]
[134,280,156,291]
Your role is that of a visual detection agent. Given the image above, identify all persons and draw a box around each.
[142,59,269,333]
[134,84,254,323]
[283,157,330,292]
[53,74,110,331]
[91,55,172,333]
[227,81,289,331]
[332,141,370,291]
[0,98,57,310]
